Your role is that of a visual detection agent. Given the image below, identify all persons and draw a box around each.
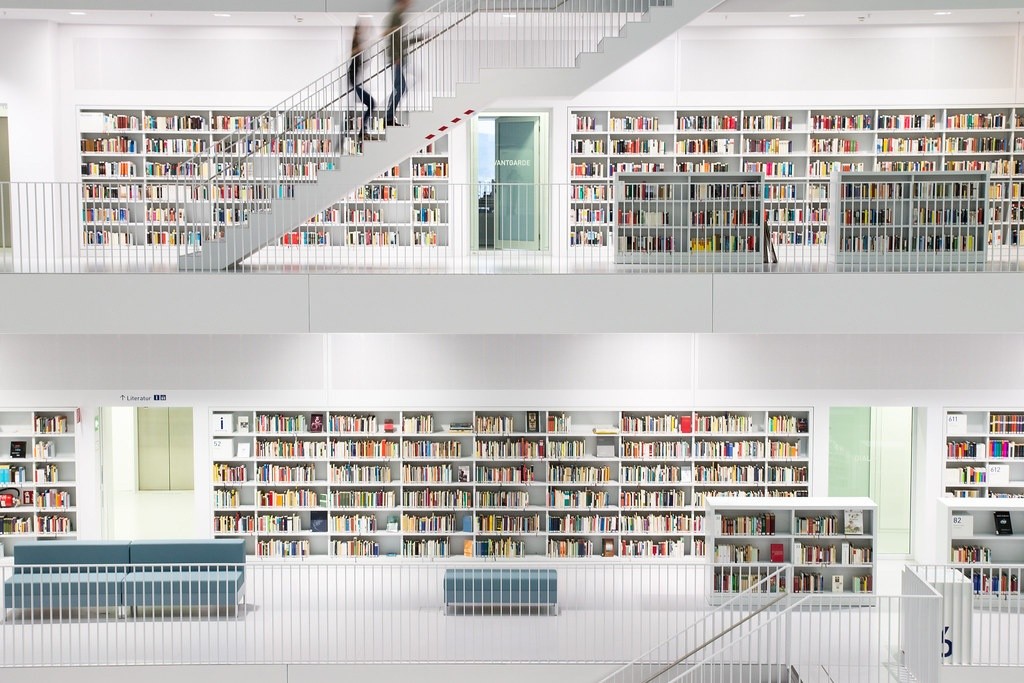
[380,0,432,127]
[333,17,382,153]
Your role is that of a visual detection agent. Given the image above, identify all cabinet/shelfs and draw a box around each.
[937,496,1024,608]
[942,406,1024,498]
[566,105,1024,255]
[704,496,878,606]
[75,106,455,260]
[827,170,990,264]
[612,170,765,265]
[207,406,814,566]
[0,408,84,541]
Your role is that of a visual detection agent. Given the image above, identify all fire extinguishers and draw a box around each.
[0,487,21,509]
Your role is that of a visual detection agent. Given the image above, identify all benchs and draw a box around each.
[3,539,132,622]
[121,538,247,617]
[443,568,558,616]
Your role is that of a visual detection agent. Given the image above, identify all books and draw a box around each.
[0,413,76,532]
[945,411,1024,499]
[840,183,984,250]
[571,114,1024,178]
[82,112,447,246]
[213,411,400,556]
[764,185,830,245]
[620,414,809,555]
[570,180,760,253]
[402,414,619,557]
[988,182,1024,246]
[714,513,872,593]
[951,511,1024,593]
